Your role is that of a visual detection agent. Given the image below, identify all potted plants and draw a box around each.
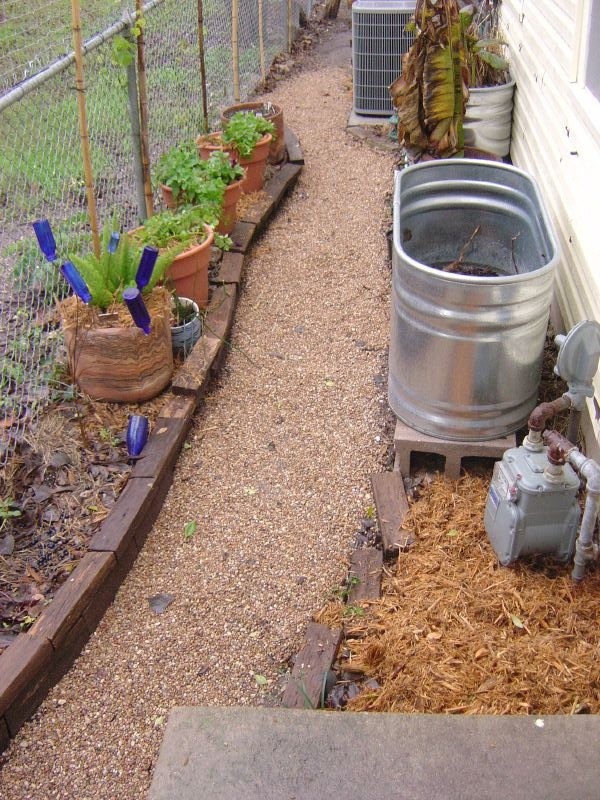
[222,103,284,163]
[152,143,247,235]
[195,114,277,194]
[387,0,514,166]
[55,218,174,400]
[128,210,213,306]
[168,289,201,350]
